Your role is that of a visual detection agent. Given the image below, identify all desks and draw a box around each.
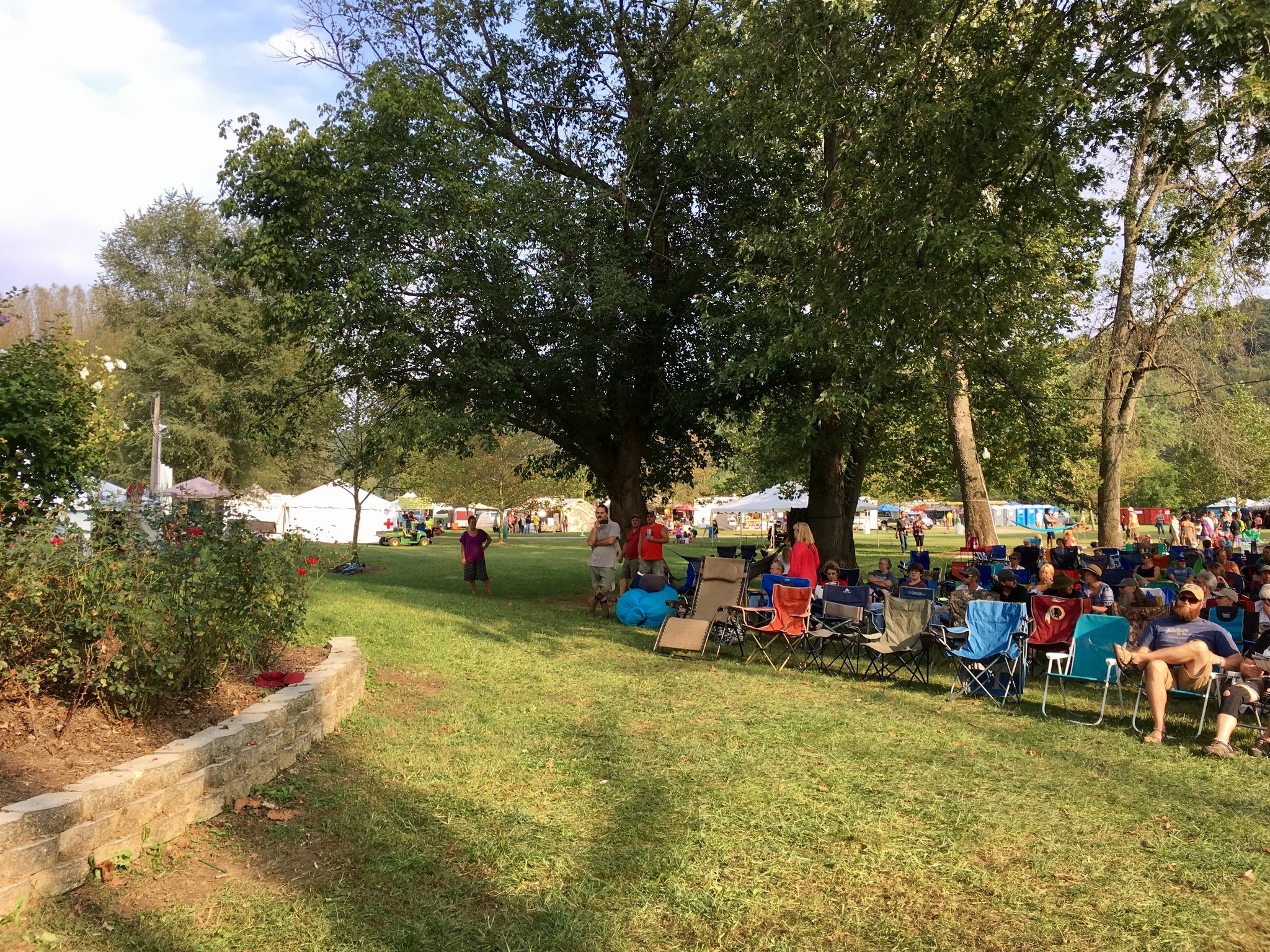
[776,534,791,547]
[539,524,561,532]
[736,534,769,548]
[1063,523,1088,533]
[432,525,442,535]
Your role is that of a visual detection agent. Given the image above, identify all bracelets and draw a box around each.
[1217,656,1222,666]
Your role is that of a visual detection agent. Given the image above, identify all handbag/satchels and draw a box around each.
[511,520,515,524]
[713,616,743,645]
[518,518,522,525]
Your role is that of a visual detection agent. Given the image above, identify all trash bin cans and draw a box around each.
[709,526,716,538]
[1120,508,1171,525]
[499,525,508,539]
[955,525,964,534]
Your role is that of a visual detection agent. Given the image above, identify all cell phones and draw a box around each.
[599,519,609,525]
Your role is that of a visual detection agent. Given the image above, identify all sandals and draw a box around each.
[1113,643,1139,672]
[1143,728,1167,743]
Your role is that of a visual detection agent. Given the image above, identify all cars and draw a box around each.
[905,512,934,530]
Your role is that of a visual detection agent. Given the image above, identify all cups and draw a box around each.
[647,528,652,540]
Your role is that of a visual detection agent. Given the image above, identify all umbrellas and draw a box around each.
[677,505,700,524]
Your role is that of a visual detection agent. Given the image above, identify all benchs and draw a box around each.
[394,527,412,537]
[767,540,791,543]
[433,531,445,534]
[1064,527,1089,530]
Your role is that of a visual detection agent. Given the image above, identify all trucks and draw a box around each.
[374,521,430,547]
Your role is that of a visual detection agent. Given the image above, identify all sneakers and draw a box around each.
[1248,736,1270,757]
[1201,737,1235,758]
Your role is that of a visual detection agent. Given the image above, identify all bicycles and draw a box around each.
[327,551,366,577]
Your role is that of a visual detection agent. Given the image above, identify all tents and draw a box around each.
[852,500,900,546]
[391,491,597,532]
[51,477,399,545]
[1206,496,1270,529]
[710,480,809,548]
[923,505,955,523]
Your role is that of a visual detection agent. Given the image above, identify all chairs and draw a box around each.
[734,536,1270,740]
[716,545,736,558]
[451,523,461,533]
[653,556,747,660]
[664,561,697,609]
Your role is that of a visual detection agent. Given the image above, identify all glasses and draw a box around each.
[1177,560,1186,562]
[910,570,919,572]
[962,574,974,578]
[1010,557,1017,560]
[1178,595,1201,604]
[1214,596,1228,600]
[647,516,655,518]
[1119,587,1124,589]
[878,562,887,565]
[1261,598,1270,601]
[797,522,800,528]
[1141,557,1148,559]
[828,572,835,575]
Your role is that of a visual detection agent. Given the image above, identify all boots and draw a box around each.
[588,595,599,616]
[599,603,610,619]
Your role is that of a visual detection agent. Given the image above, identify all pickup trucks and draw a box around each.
[878,510,901,531]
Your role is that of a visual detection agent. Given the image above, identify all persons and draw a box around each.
[587,504,669,620]
[563,515,568,533]
[894,510,965,556]
[507,511,540,534]
[459,516,494,595]
[397,510,444,545]
[1114,583,1245,744]
[768,524,786,548]
[1035,505,1270,547]
[1201,629,1270,759]
[769,521,1270,642]
[655,513,720,544]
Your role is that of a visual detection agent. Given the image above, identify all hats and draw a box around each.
[1179,583,1204,601]
[646,510,656,517]
[1261,565,1270,572]
[998,569,1020,582]
[1078,564,1102,578]
[1210,588,1238,603]
[1065,530,1074,540]
[959,567,980,579]
[1115,578,1140,588]
[1127,507,1134,511]
[1174,554,1185,561]
[1191,541,1198,548]
[632,514,641,520]
[953,509,956,511]
[1051,571,1076,588]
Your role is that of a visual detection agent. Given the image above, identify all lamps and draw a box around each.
[977,447,1000,546]
[158,424,167,501]
